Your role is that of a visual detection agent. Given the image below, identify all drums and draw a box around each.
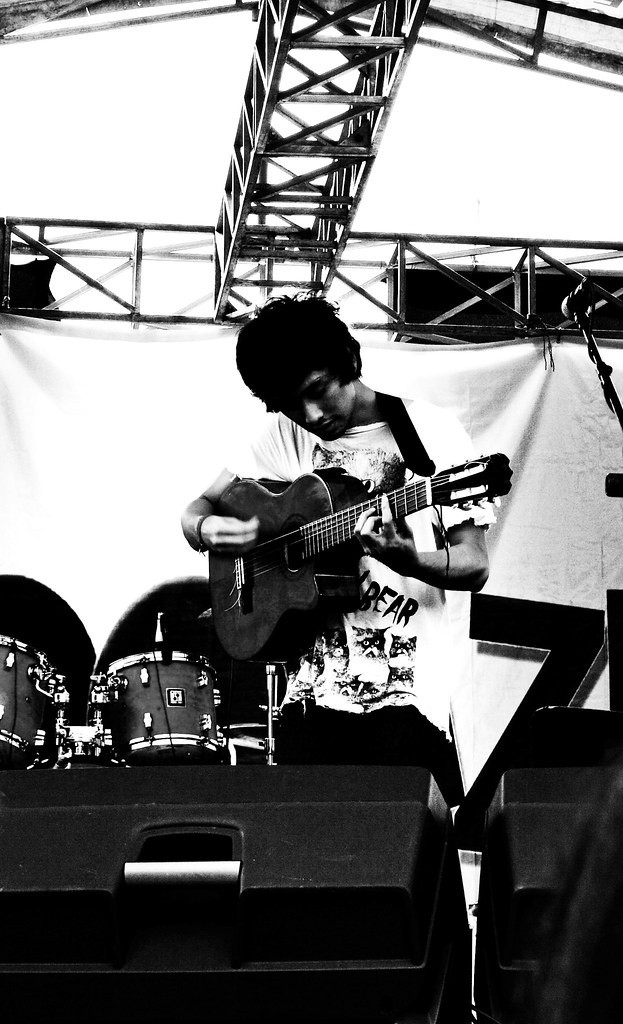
[61,725,106,768]
[106,649,221,765]
[0,634,51,763]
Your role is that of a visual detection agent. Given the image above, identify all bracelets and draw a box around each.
[198,515,212,557]
[444,546,450,588]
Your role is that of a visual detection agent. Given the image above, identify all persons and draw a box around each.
[180,292,496,1024]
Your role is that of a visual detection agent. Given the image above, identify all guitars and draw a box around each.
[208,450,514,663]
